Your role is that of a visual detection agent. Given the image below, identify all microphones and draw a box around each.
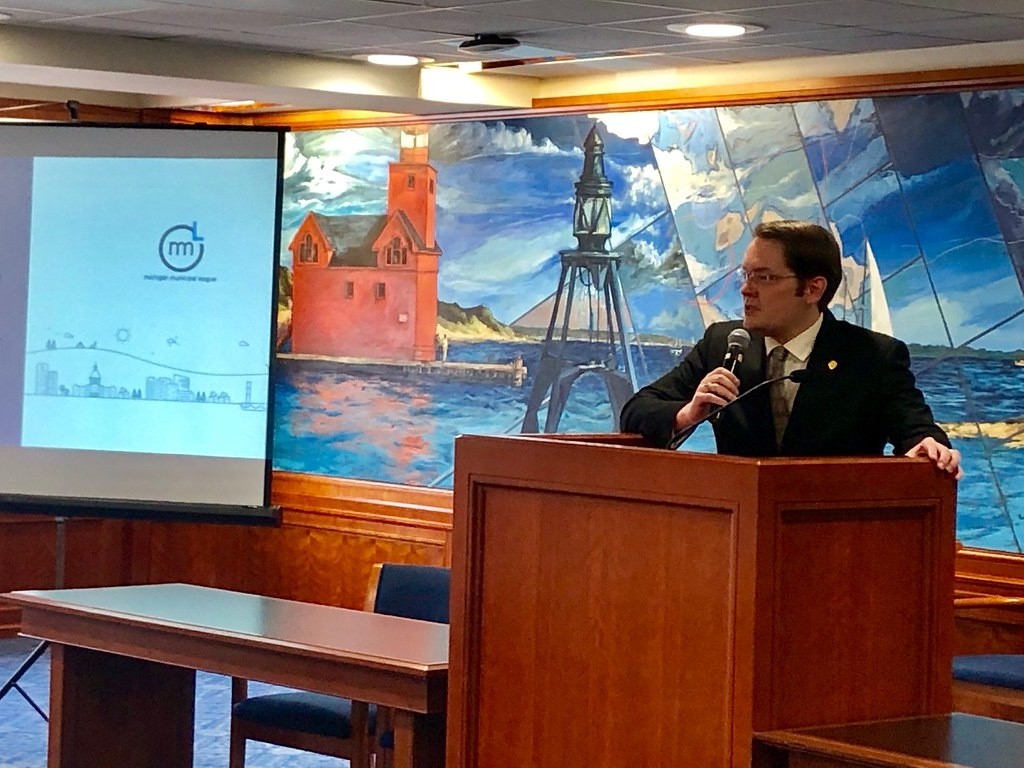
[707,328,750,424]
[663,369,813,449]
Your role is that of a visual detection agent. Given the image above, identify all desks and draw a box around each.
[0,583,450,768]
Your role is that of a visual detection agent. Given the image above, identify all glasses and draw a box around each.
[736,269,797,283]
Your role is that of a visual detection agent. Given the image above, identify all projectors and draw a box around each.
[459,38,519,53]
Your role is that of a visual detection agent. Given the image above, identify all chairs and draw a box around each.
[951,655,1024,723]
[229,562,451,768]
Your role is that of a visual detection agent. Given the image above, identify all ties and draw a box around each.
[769,347,790,448]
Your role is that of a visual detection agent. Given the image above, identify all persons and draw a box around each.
[619,220,963,482]
